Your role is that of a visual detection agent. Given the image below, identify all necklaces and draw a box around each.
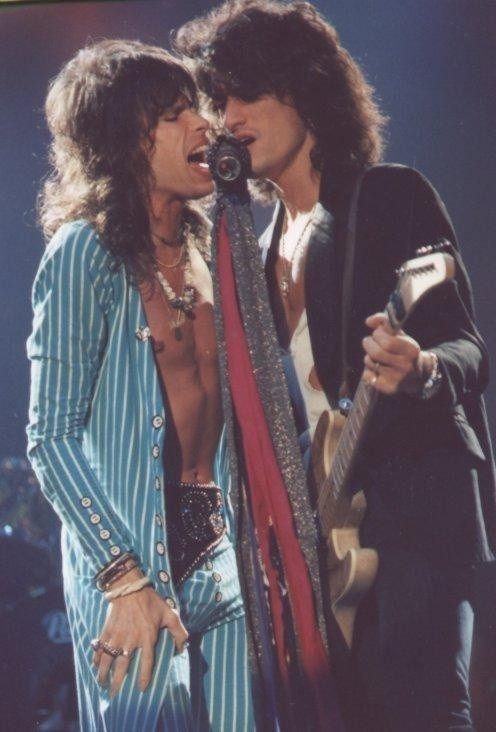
[279,205,313,301]
[137,223,198,343]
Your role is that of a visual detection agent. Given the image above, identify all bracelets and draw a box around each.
[420,351,443,404]
[90,549,152,601]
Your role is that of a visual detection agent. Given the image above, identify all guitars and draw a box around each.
[305,241,455,672]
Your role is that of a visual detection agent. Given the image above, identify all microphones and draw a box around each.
[215,141,243,182]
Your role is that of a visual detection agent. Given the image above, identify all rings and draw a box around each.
[371,376,378,386]
[101,641,117,658]
[373,361,380,374]
[118,647,133,659]
[89,639,100,650]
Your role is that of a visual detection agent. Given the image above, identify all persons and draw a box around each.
[24,35,275,732]
[171,0,496,730]
